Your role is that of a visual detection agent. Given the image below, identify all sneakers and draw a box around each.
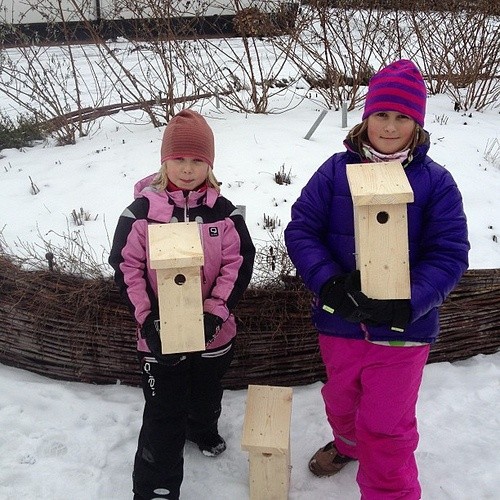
[307,440,358,479]
[186,430,228,458]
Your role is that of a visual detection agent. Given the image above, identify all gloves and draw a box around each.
[139,309,188,368]
[192,311,224,355]
[318,270,361,315]
[348,290,412,334]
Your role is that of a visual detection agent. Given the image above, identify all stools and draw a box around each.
[147,221,206,356]
[241,384,293,500]
[345,162,414,300]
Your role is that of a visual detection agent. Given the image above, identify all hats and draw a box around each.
[160,108,216,170]
[361,59,428,130]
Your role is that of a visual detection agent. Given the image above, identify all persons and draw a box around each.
[105,108,256,500]
[282,57,470,500]
